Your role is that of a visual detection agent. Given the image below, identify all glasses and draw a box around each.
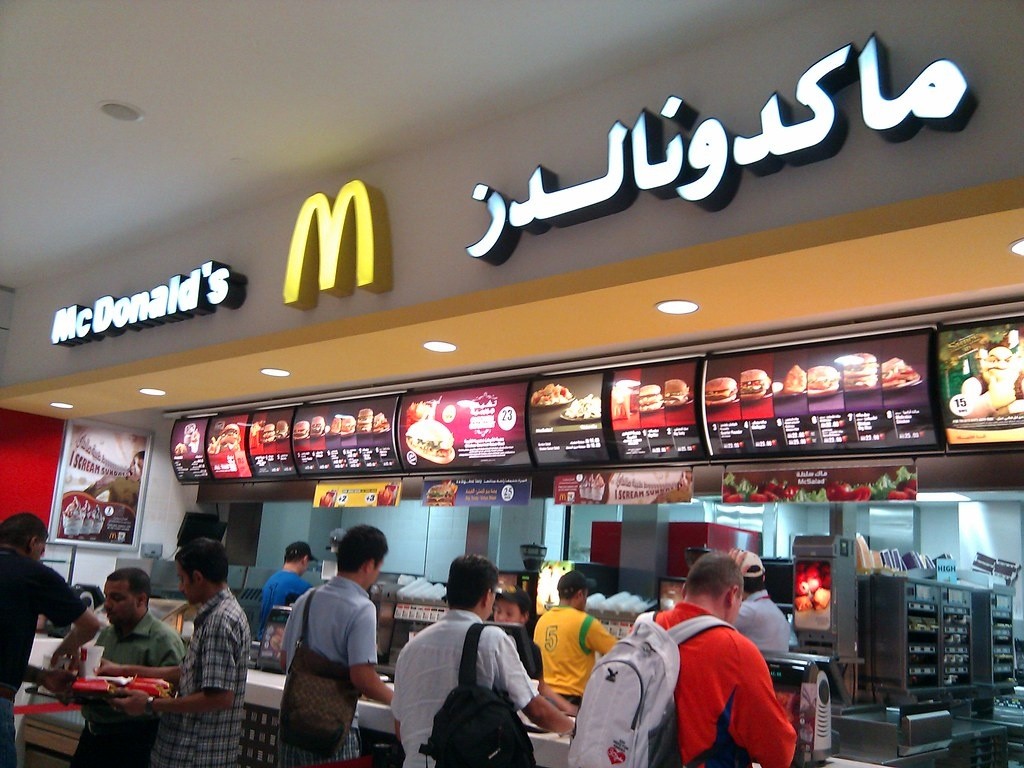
[496,586,526,601]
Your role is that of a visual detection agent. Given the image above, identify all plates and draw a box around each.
[531,397,576,404]
[559,409,600,421]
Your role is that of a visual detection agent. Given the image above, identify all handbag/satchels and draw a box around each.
[280,647,361,758]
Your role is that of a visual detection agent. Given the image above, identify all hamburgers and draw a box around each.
[263,409,376,445]
[427,484,454,506]
[705,369,768,408]
[404,418,456,464]
[218,423,241,452]
[638,379,691,412]
[807,353,880,397]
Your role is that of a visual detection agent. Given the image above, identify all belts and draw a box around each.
[85,719,160,737]
[0,688,15,702]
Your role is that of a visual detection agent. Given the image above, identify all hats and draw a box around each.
[735,552,766,577]
[557,570,597,592]
[286,542,316,561]
[496,586,530,611]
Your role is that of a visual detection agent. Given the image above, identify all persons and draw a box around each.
[630,553,798,768]
[0,512,100,768]
[70,567,186,768]
[727,547,790,653]
[530,570,620,706]
[88,537,251,768]
[276,526,395,768]
[391,555,577,768]
[258,542,320,645]
[491,584,578,715]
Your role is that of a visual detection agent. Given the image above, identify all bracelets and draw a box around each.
[31,667,44,682]
[144,696,155,720]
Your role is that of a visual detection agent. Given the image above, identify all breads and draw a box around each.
[881,357,919,388]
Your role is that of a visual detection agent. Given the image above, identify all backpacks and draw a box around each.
[565,611,743,768]
[418,624,536,768]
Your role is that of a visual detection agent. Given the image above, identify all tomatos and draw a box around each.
[795,560,830,596]
[722,478,914,504]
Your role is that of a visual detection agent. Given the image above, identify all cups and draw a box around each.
[585,488,593,500]
[62,516,83,535]
[592,487,605,501]
[79,646,105,679]
[580,489,586,498]
[90,523,103,534]
[80,521,94,534]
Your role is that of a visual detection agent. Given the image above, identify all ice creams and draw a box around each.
[63,496,104,534]
